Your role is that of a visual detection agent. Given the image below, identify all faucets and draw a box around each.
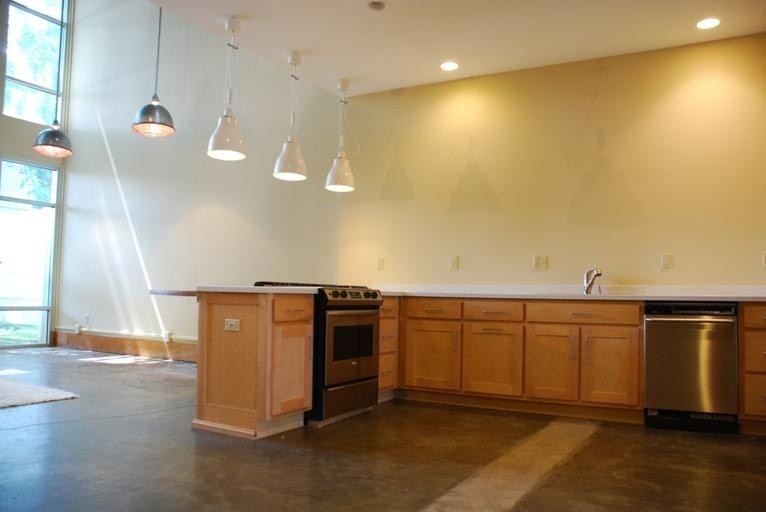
[583,267,602,295]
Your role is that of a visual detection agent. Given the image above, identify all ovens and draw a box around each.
[309,308,383,421]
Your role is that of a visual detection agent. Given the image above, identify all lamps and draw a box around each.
[207,16,247,161]
[131,5,178,139]
[324,79,357,194]
[273,49,310,183]
[33,0,75,159]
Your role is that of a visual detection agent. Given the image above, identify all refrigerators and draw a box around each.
[643,300,740,436]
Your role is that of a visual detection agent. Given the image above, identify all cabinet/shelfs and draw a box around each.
[738,301,766,425]
[523,295,643,427]
[395,292,462,403]
[378,292,401,405]
[191,288,315,439]
[463,297,522,412]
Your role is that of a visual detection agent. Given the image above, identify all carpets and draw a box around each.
[1,375,79,413]
